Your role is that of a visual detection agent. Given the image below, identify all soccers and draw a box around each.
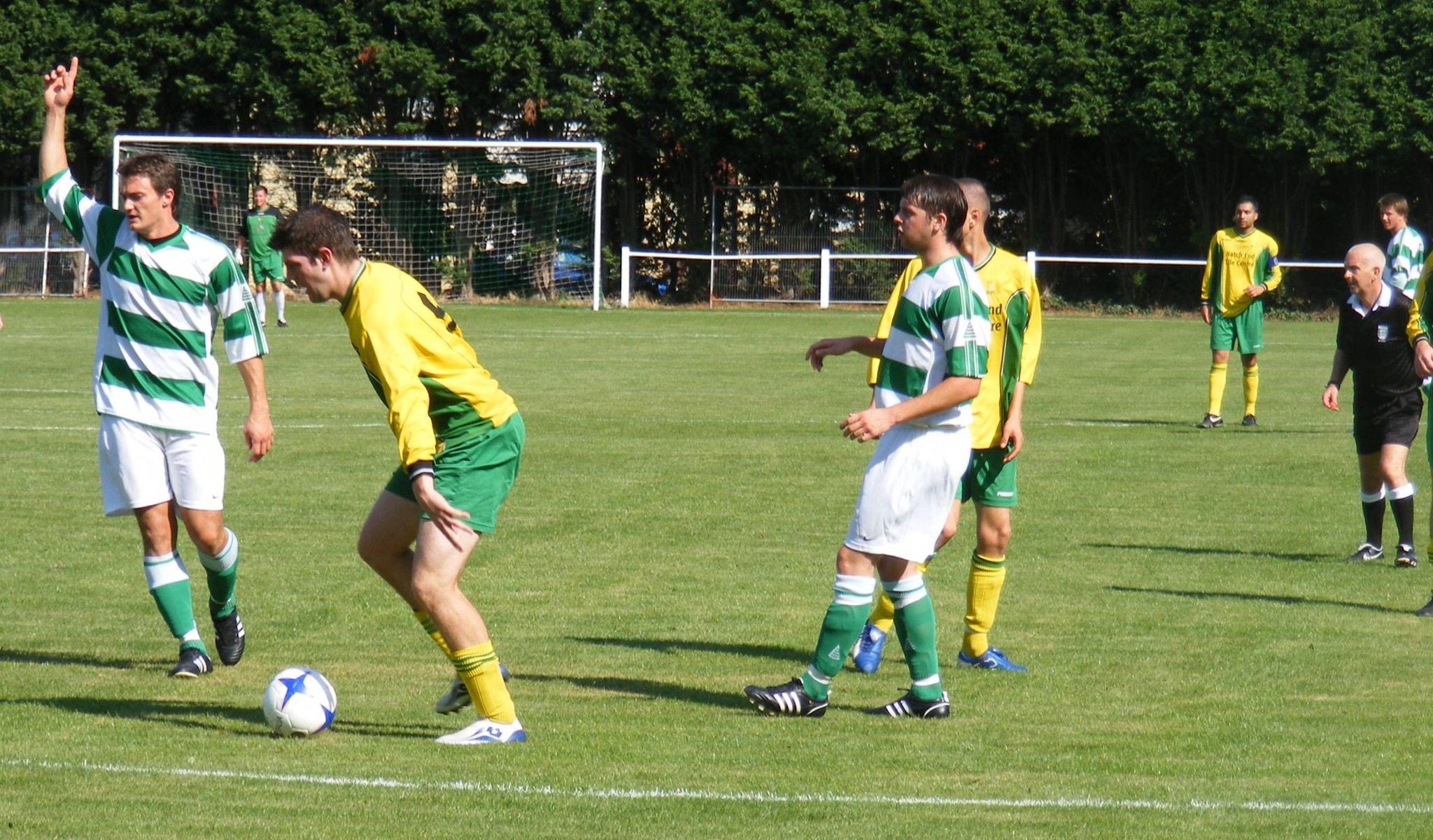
[262,667,337,739]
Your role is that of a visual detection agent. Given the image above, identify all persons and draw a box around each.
[840,177,1041,675]
[267,202,526,748]
[1322,243,1423,568]
[743,173,991,718]
[1197,195,1282,429]
[1406,249,1433,619]
[39,56,275,679]
[234,185,290,328]
[1379,194,1426,302]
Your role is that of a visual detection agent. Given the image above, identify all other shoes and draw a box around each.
[260,321,266,328]
[277,319,289,328]
[1415,589,1433,617]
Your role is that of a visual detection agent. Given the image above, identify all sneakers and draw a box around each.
[1346,543,1385,561]
[434,717,527,745]
[851,618,887,674]
[209,599,245,666]
[1198,413,1224,428]
[168,647,213,679]
[435,661,511,715]
[957,647,1028,673]
[1395,542,1418,567]
[862,688,950,719]
[742,677,832,718]
[1242,413,1259,427]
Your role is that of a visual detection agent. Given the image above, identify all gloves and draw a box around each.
[234,248,243,265]
